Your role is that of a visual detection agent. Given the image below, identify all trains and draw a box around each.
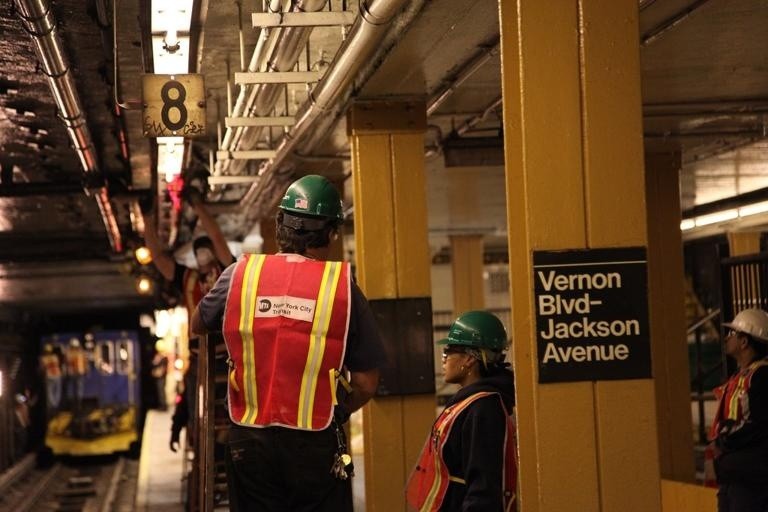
[36,328,145,458]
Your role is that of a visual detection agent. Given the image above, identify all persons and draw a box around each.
[705,309,768,512]
[405,310,517,512]
[136,190,236,317]
[29,332,199,455]
[191,172,381,512]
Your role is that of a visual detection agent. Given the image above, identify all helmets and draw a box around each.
[437,310,506,350]
[720,309,768,341]
[277,175,343,219]
